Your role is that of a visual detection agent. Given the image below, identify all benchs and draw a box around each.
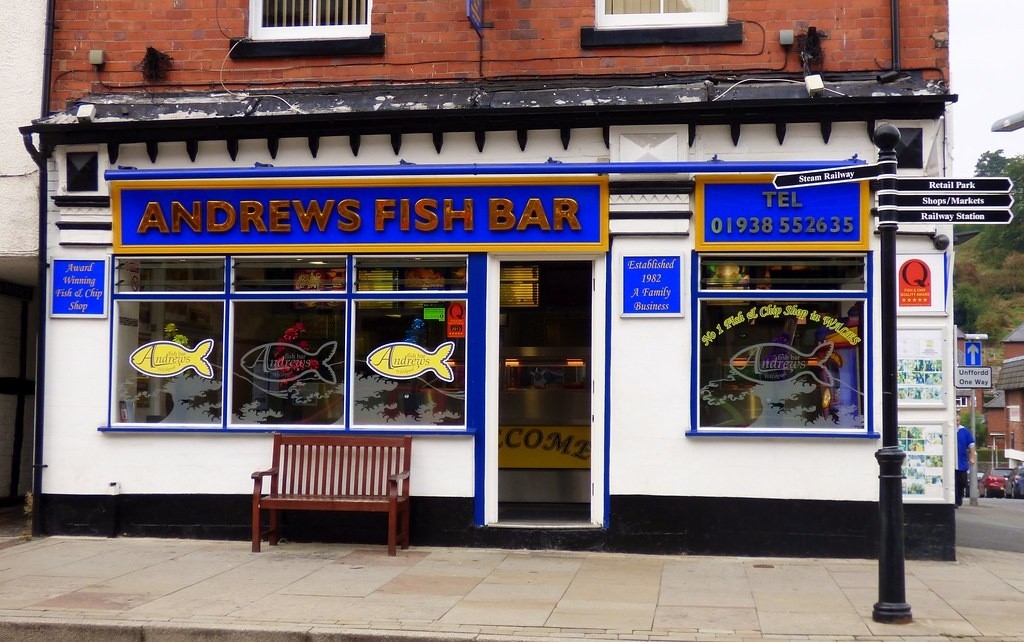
[250,432,412,557]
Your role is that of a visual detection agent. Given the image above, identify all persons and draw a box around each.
[954,414,975,508]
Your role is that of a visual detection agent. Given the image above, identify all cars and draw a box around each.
[1003,466,1024,499]
[978,466,1015,498]
[965,472,986,498]
[1015,476,1024,499]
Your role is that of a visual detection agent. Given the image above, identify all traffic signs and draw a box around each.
[894,190,1015,209]
[895,208,1015,225]
[771,163,880,190]
[895,177,1015,194]
